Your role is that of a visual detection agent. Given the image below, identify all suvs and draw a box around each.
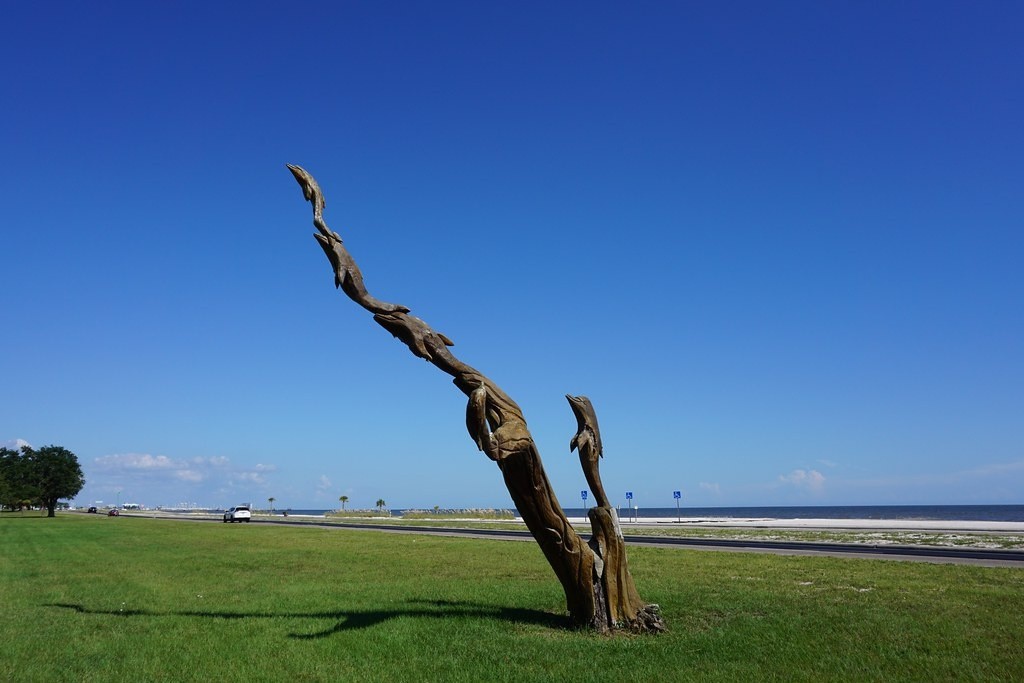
[223,505,252,524]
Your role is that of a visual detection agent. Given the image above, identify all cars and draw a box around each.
[108,509,120,517]
[88,506,97,513]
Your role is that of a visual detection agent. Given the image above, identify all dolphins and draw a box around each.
[286,161,500,461]
[565,393,611,511]
